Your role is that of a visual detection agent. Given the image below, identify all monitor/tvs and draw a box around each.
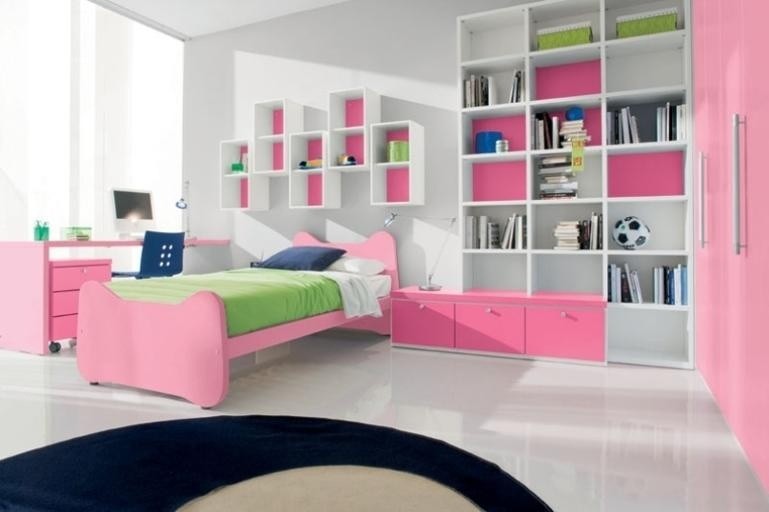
[113,189,154,239]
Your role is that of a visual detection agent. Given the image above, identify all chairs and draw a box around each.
[112,230,185,279]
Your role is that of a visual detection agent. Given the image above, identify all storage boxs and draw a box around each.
[615,7,678,39]
[536,21,593,50]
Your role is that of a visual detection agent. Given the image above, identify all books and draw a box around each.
[607,101,687,146]
[464,68,525,109]
[466,213,527,249]
[531,112,591,199]
[608,261,686,307]
[552,211,603,250]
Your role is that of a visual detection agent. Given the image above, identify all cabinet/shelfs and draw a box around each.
[456,0,695,370]
[49,259,113,353]
[219,88,425,213]
[390,284,608,366]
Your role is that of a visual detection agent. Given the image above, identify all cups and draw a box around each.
[496,139,509,154]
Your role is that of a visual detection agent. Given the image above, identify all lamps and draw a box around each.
[176,181,191,238]
[383,209,456,291]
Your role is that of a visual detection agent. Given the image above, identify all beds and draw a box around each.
[76,230,400,409]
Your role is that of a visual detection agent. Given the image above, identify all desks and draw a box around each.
[0,238,230,355]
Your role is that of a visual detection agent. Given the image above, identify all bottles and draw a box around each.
[33,219,50,241]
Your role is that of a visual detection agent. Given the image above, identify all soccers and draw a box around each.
[613,217,651,248]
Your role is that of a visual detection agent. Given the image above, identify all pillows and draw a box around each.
[322,256,385,276]
[257,245,348,271]
